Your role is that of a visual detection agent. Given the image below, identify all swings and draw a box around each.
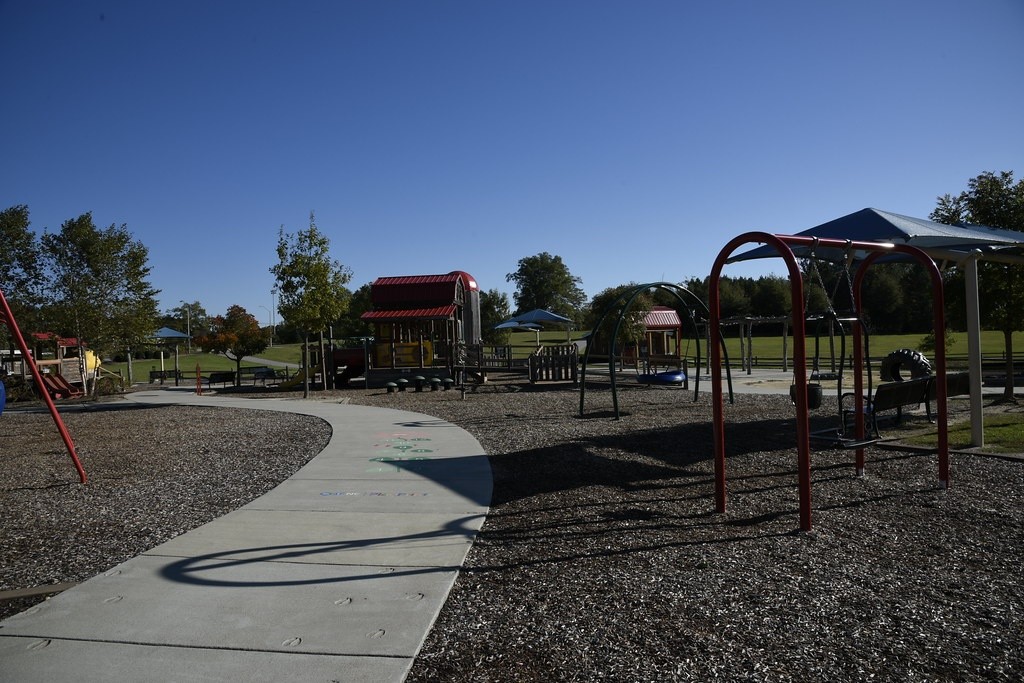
[620,316,693,385]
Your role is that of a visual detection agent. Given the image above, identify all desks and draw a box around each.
[384,343,431,366]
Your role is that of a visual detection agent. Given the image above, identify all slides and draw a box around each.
[42,373,80,396]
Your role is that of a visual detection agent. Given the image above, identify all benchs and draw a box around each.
[841,372,969,437]
[240,365,268,378]
[196,370,237,389]
[249,378,282,387]
[149,370,181,383]
